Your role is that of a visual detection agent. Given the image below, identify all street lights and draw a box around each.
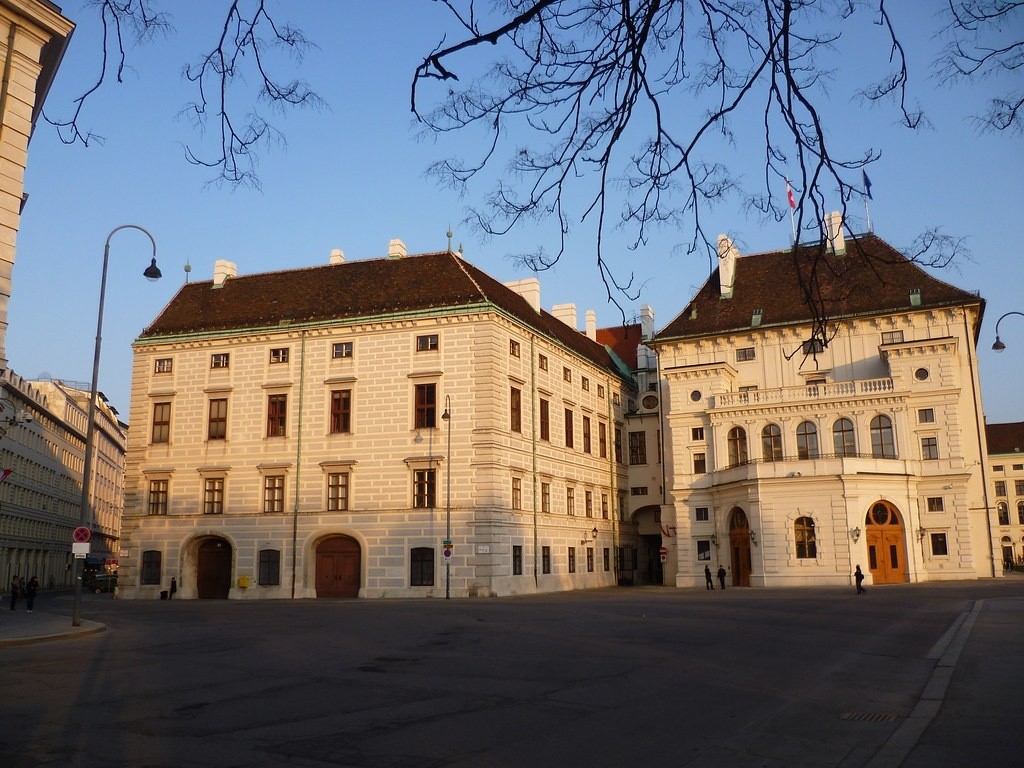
[443,396,451,599]
[1012,542,1017,567]
[73,225,162,626]
[992,312,1024,354]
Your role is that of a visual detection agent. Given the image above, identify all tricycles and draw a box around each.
[1004,557,1014,572]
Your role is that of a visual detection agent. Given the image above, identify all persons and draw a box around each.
[18,576,26,600]
[50,575,54,590]
[26,576,38,614]
[11,575,20,610]
[168,577,177,600]
[717,565,726,589]
[705,565,715,590]
[854,565,865,594]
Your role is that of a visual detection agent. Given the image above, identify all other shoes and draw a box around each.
[27,609,32,614]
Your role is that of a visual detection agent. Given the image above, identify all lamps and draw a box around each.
[748,531,757,546]
[581,527,599,546]
[918,527,925,540]
[854,527,862,543]
[710,534,716,543]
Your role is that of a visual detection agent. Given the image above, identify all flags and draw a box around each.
[863,169,873,200]
[786,178,795,208]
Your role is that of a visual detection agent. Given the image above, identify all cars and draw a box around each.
[92,578,116,594]
[96,574,117,578]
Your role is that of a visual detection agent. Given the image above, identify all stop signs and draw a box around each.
[660,548,666,555]
